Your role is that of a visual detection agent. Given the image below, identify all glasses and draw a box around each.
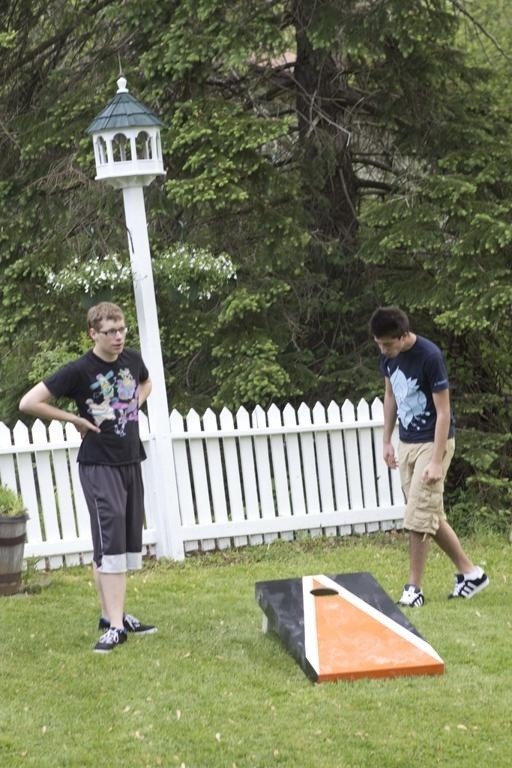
[98,327,126,337]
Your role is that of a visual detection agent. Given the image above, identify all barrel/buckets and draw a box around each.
[0,514,28,594]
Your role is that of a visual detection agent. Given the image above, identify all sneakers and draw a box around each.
[98,613,158,638]
[393,583,426,608]
[91,625,128,653]
[448,565,491,600]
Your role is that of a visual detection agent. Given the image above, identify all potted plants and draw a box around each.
[0,483,31,595]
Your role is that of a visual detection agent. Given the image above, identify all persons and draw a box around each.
[18,299,160,655]
[367,304,490,607]
